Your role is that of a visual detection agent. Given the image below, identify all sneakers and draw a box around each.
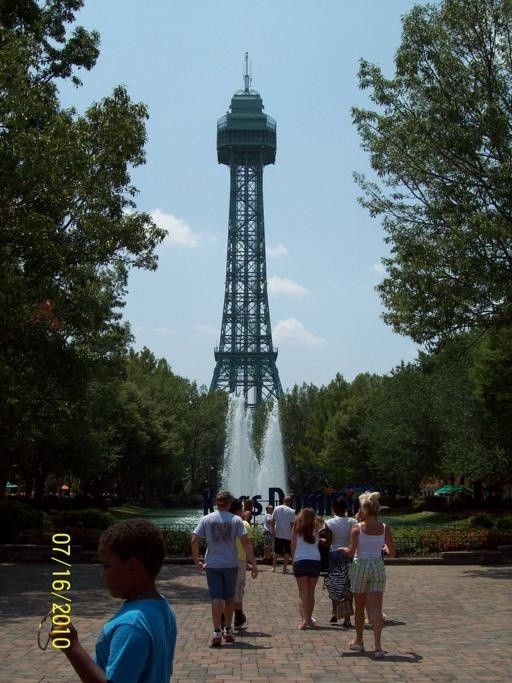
[329,616,337,622]
[374,648,384,658]
[213,613,246,646]
[348,639,364,651]
[343,622,354,628]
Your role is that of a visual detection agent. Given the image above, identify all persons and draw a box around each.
[337,491,396,659]
[263,505,274,561]
[291,507,321,629]
[242,511,252,524]
[189,490,259,644]
[324,497,359,626]
[270,496,296,574]
[49,518,178,683]
[219,499,257,632]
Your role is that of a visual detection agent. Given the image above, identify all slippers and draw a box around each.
[298,618,316,630]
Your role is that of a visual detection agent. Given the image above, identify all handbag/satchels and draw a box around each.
[333,600,354,619]
